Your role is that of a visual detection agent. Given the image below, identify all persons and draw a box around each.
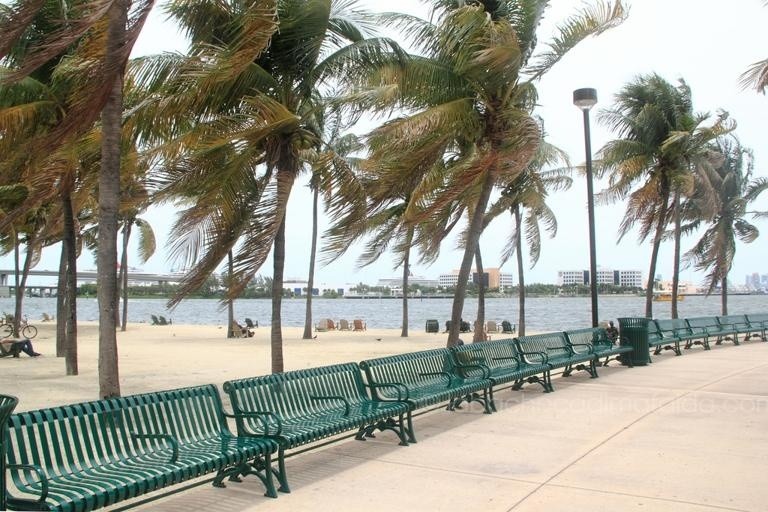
[0,336,41,357]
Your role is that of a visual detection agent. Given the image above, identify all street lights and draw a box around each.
[573,87,600,367]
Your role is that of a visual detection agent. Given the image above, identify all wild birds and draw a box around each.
[376,338,381,341]
[314,335,317,339]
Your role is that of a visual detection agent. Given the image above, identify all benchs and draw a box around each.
[449,338,550,413]
[358,347,493,443]
[223,362,410,494]
[563,328,634,377]
[4,383,277,512]
[645,314,768,356]
[513,332,597,392]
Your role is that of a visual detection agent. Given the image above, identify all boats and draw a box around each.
[653,291,684,301]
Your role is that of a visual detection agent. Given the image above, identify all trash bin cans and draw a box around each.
[617,317,651,366]
[0,394,18,511]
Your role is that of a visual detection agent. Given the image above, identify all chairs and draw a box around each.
[425,319,516,334]
[315,318,367,331]
[151,315,172,325]
[232,318,258,338]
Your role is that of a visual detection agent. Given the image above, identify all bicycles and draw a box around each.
[0,317,38,339]
